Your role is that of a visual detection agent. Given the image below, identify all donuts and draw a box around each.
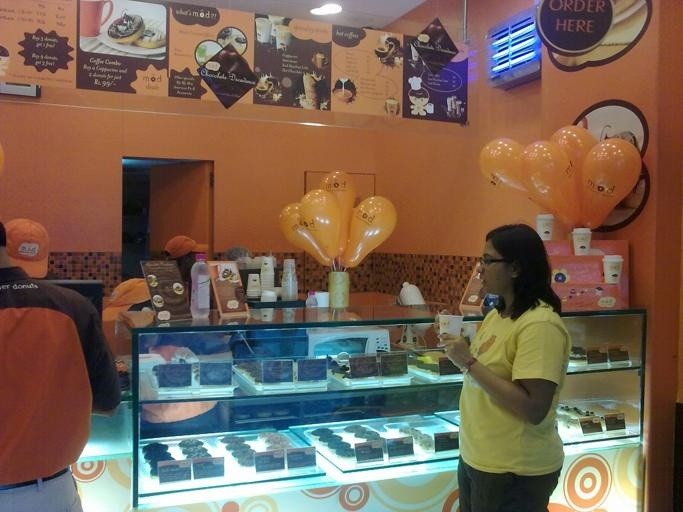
[172,346,198,364]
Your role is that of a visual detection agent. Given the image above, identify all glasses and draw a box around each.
[478,257,504,265]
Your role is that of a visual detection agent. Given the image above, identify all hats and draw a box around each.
[164,235,209,259]
[101,277,151,321]
[5,218,48,279]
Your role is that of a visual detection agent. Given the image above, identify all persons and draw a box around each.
[0,222,121,512]
[165,237,209,282]
[433,224,572,512]
[100,278,221,439]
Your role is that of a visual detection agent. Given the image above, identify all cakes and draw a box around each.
[570,347,587,365]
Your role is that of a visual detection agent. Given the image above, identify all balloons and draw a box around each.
[341,195,397,269]
[479,138,531,197]
[279,203,334,267]
[320,171,355,239]
[548,125,598,176]
[298,189,348,260]
[581,138,642,231]
[516,141,584,229]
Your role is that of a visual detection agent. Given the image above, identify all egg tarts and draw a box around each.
[134,25,167,49]
[107,14,145,44]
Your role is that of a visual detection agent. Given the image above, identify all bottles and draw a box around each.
[191,253,210,317]
[305,291,317,307]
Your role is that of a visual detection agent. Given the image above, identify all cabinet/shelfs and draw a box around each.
[130,304,648,512]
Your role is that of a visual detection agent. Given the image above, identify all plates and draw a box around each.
[233,364,327,391]
[328,370,411,386]
[148,371,237,397]
[408,364,466,381]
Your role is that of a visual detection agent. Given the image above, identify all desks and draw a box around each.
[61,354,134,463]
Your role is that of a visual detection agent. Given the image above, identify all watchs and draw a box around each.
[458,358,476,375]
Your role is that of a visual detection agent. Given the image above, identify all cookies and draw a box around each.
[179,439,211,460]
[220,435,257,466]
[310,428,355,458]
[400,427,435,452]
[344,424,386,448]
[143,442,176,476]
[260,430,293,452]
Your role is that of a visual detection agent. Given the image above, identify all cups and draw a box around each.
[536,214,554,240]
[603,257,622,284]
[247,274,261,297]
[572,229,592,255]
[282,259,298,300]
[261,257,274,292]
[439,314,463,338]
